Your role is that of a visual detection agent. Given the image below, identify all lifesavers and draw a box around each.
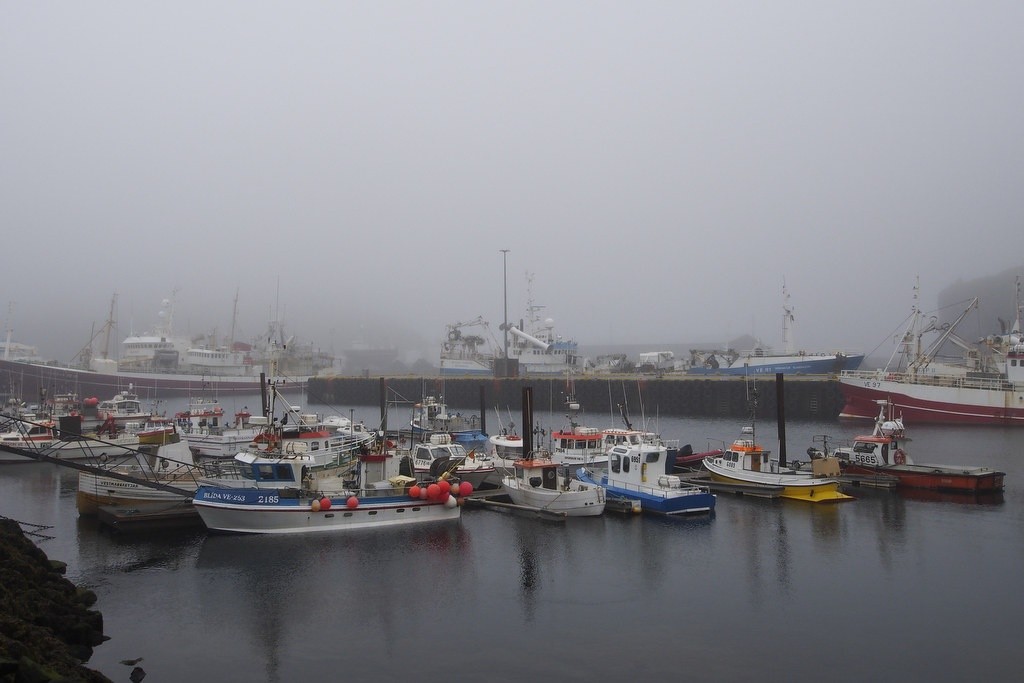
[894,449,905,465]
[508,436,521,440]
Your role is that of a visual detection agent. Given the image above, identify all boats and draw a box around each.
[438,268,866,373]
[837,273,1024,424]
[805,411,1006,492]
[77,428,253,531]
[575,437,717,516]
[377,392,725,489]
[501,449,607,516]
[702,422,853,503]
[190,417,460,534]
[177,382,266,456]
[0,292,340,401]
[0,385,173,461]
